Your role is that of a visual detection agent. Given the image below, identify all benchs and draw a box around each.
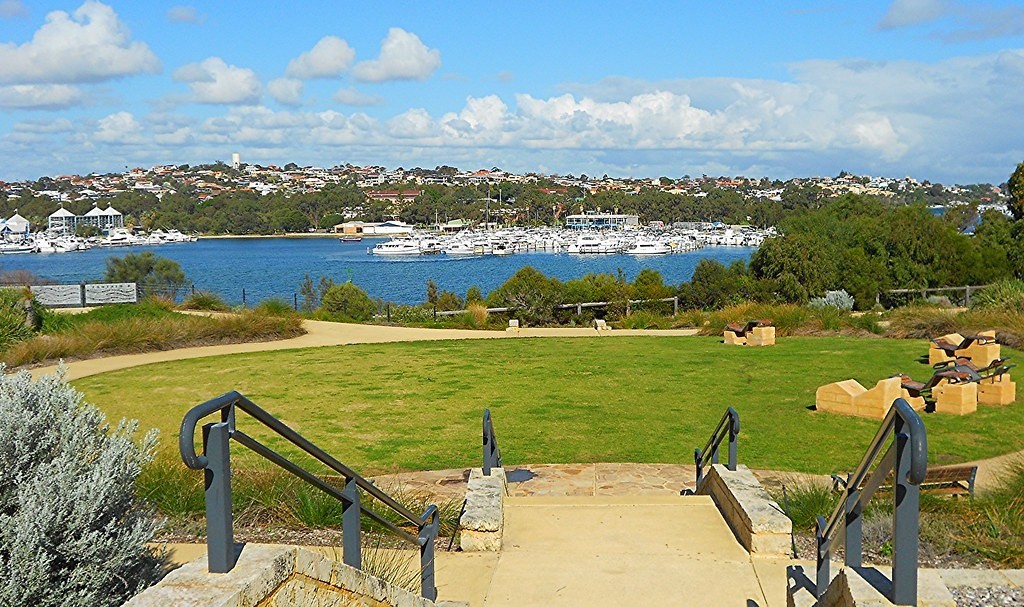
[830,465,978,501]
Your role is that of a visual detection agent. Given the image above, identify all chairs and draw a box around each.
[726,319,772,337]
[889,366,973,397]
[930,334,999,352]
[933,357,1011,374]
[955,364,1016,384]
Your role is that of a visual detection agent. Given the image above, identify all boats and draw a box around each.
[371,227,783,256]
[0,228,199,254]
[339,234,362,243]
